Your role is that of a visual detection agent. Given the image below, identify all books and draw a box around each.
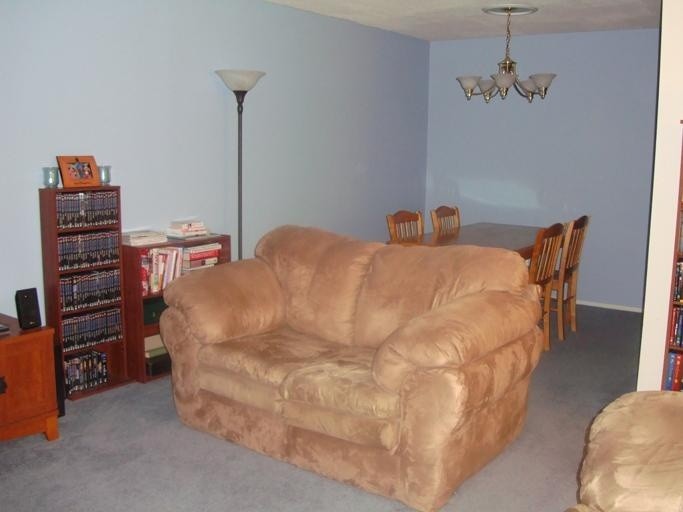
[54,190,223,397]
[664,263,683,391]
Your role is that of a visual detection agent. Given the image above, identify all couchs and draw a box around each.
[565,390,683,512]
[159,224,543,512]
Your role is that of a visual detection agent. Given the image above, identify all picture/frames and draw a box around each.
[57,156,101,188]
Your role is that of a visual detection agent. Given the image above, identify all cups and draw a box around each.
[42,167,60,189]
[98,165,111,186]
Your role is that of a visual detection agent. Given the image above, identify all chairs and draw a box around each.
[430,205,460,232]
[529,223,564,351]
[387,210,424,239]
[552,215,589,341]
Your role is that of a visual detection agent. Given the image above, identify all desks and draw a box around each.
[386,222,565,260]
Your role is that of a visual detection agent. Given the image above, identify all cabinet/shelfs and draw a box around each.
[122,233,231,383]
[39,186,128,400]
[661,119,683,391]
[1,312,59,444]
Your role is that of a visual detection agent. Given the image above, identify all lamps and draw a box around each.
[456,3,557,104]
[214,68,266,261]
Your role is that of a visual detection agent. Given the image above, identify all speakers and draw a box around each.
[15,288,41,329]
